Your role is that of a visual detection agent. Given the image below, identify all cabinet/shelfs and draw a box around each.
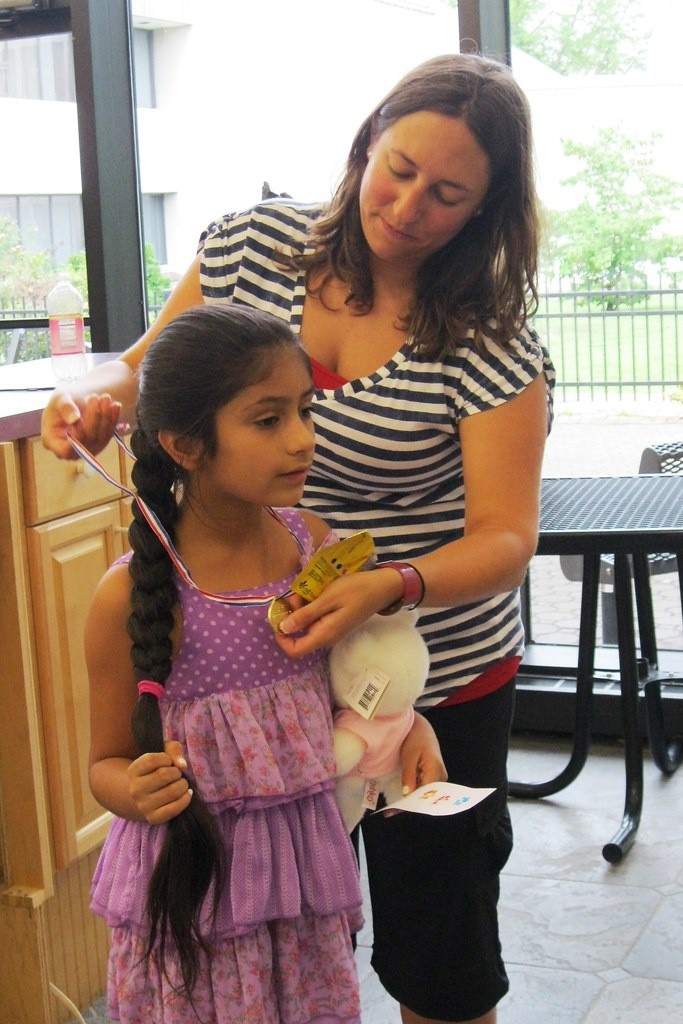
[0,351,184,1024]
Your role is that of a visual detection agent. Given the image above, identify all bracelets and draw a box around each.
[368,561,425,616]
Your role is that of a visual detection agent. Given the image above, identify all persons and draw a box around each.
[42,52,555,1024]
[84,305,447,1024]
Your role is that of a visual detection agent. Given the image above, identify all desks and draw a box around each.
[505,474,683,866]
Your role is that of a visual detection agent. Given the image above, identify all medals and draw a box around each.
[267,599,295,636]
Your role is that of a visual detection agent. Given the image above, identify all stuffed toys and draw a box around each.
[326,604,430,834]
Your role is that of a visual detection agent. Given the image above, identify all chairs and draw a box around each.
[558,440,683,645]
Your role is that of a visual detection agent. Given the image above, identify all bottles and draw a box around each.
[47,271,86,356]
